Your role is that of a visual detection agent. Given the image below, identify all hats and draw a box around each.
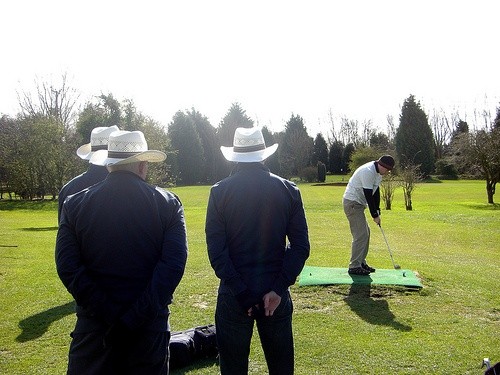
[89,130,167,166]
[378,156,395,170]
[220,127,279,162]
[76,125,120,160]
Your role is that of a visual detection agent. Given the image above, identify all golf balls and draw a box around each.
[402,270,406,273]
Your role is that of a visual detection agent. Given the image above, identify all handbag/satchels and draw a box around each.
[169,324,219,375]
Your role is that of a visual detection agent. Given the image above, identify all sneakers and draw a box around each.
[361,262,375,272]
[348,267,370,275]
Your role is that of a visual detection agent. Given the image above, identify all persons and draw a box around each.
[342,155,395,275]
[58,125,120,225]
[55,130,188,375]
[206,126,310,375]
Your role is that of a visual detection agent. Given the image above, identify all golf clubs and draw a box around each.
[378,224,401,270]
